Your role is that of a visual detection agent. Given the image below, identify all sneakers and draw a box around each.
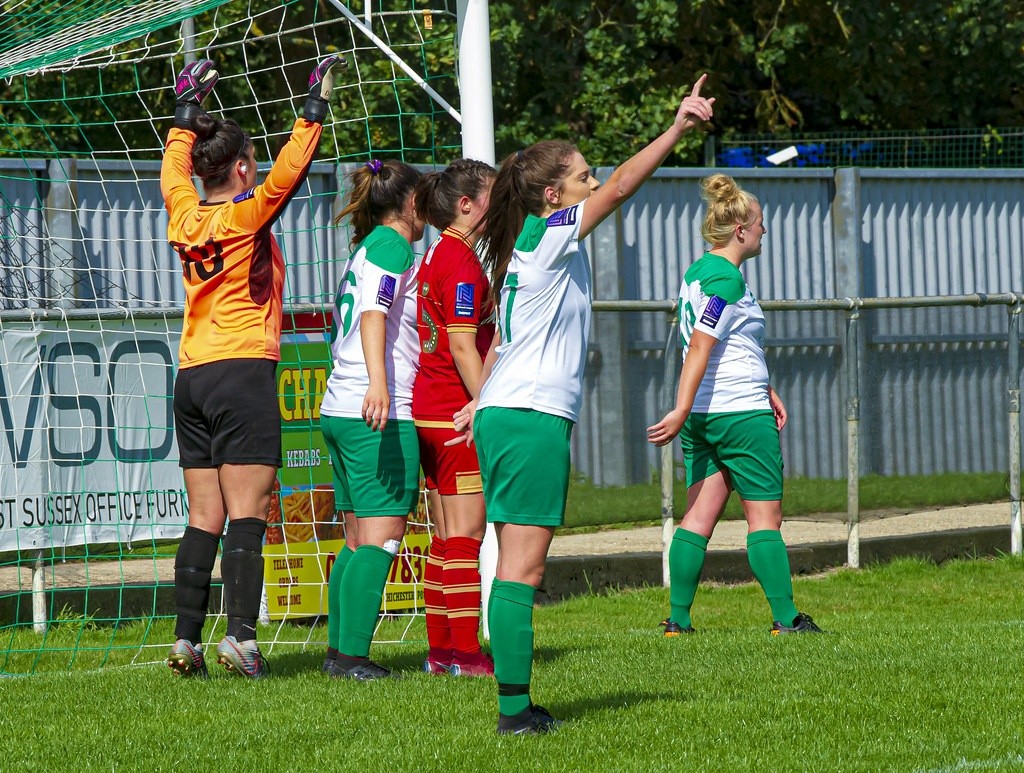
[331,652,400,681]
[167,639,209,676]
[449,653,494,676]
[323,647,337,673]
[496,702,563,736]
[424,656,451,675]
[771,612,824,635]
[663,622,696,637]
[216,635,270,677]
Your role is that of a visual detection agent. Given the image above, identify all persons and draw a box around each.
[318,160,419,681]
[160,56,349,679]
[412,158,495,676]
[444,72,716,733]
[647,174,824,639]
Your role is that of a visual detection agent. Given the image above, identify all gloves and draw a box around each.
[302,55,350,126]
[173,59,219,129]
